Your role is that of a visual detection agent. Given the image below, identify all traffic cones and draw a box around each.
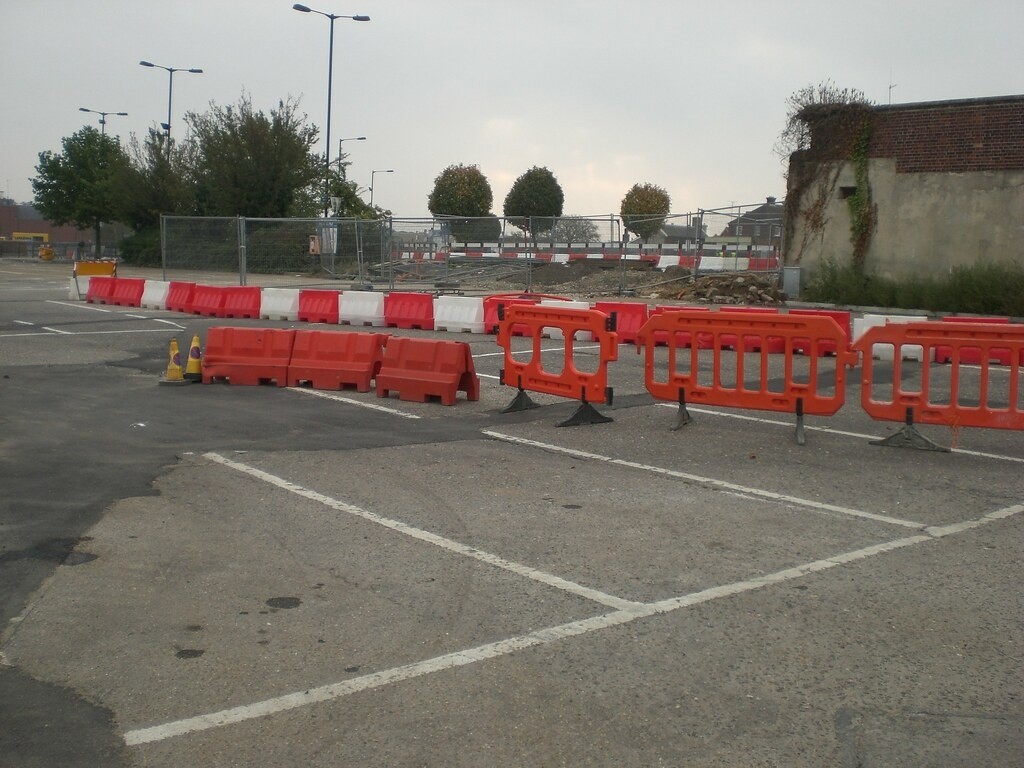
[156,337,193,385]
[182,334,201,383]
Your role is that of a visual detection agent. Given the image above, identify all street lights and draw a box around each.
[370,170,394,214]
[338,137,366,178]
[139,60,204,161]
[79,107,127,257]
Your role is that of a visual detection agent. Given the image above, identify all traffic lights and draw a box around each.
[293,3,371,218]
